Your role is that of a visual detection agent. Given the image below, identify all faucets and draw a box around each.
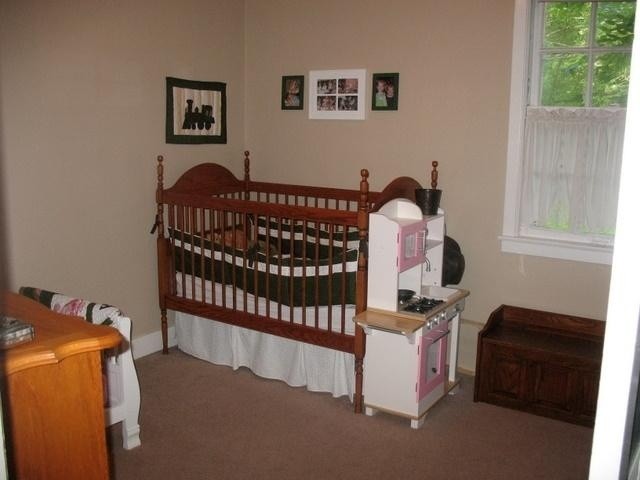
[425,257,431,272]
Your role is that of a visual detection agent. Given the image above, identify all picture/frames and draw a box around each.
[281,75,304,110]
[309,68,366,121]
[372,73,399,111]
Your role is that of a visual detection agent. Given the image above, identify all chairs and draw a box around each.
[19,284,142,450]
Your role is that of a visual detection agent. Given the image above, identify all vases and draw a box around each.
[415,189,442,216]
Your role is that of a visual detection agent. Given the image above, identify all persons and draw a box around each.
[384,84,395,107]
[375,80,388,107]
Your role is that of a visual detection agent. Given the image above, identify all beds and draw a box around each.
[155,150,439,414]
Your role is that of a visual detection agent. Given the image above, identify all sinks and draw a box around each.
[421,285,458,300]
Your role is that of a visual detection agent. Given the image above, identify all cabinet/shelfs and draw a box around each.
[473,305,606,428]
[352,197,471,429]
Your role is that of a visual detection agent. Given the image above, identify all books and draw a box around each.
[2,316,34,341]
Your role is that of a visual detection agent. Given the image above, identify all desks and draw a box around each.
[0,288,125,480]
